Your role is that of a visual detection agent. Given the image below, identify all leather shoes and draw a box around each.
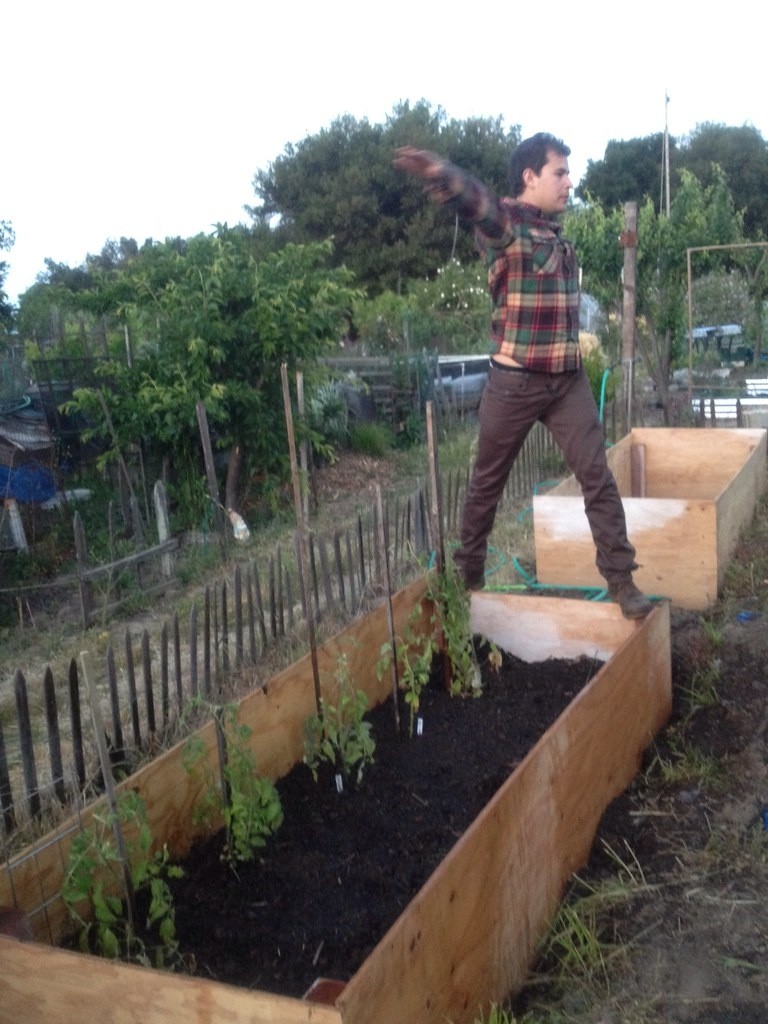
[607,573,652,619]
[450,565,487,591]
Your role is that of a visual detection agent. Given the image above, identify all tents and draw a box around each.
[686,325,742,353]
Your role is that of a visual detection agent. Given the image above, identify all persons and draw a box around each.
[395,134,652,619]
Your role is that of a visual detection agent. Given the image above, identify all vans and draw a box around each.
[432,354,495,411]
